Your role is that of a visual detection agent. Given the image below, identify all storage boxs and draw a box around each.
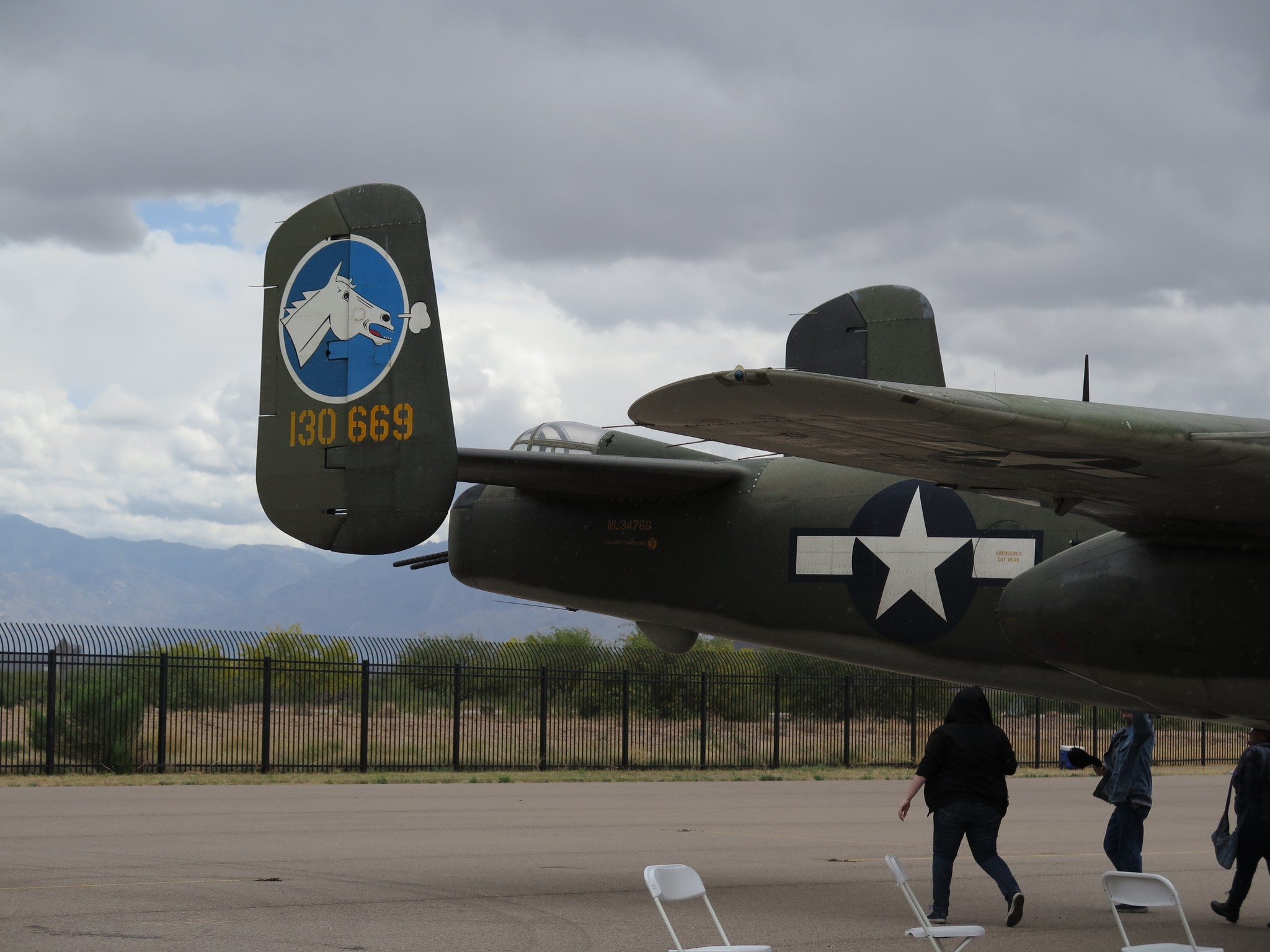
[1059,745,1085,770]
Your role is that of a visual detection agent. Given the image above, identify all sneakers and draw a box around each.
[1006,886,1024,927]
[926,905,947,923]
[1115,903,1148,913]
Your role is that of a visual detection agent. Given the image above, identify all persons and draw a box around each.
[1209,726,1270,928]
[1091,706,1157,911]
[898,687,1026,927]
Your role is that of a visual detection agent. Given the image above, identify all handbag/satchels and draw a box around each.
[1212,812,1240,869]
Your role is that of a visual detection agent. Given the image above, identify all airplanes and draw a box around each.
[255,179,1269,736]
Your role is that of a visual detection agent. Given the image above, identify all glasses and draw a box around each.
[1249,727,1256,733]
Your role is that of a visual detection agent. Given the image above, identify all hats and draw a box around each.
[1067,747,1102,769]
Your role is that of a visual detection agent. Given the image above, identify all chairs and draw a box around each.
[644,864,772,952]
[884,853,985,952]
[1102,871,1223,952]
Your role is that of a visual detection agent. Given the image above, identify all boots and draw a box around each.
[1210,891,1240,923]
